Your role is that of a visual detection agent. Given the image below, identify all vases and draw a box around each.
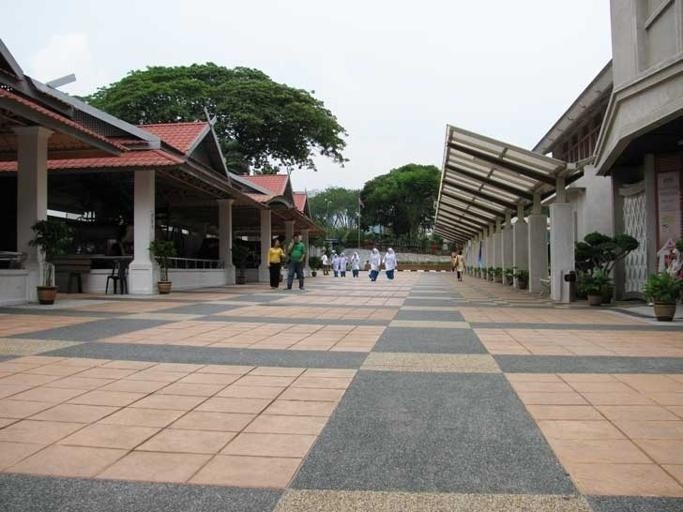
[279,274,283,282]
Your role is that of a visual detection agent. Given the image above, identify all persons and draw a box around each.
[456,251,466,281]
[320,247,397,282]
[267,239,285,288]
[283,234,306,290]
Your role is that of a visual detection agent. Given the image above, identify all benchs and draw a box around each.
[48,256,92,294]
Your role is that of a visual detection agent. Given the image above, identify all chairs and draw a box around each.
[105,260,128,295]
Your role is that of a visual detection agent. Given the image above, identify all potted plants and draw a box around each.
[147,239,178,294]
[574,231,640,306]
[640,270,683,321]
[309,255,321,277]
[477,266,529,289]
[232,242,250,284]
[28,219,67,305]
